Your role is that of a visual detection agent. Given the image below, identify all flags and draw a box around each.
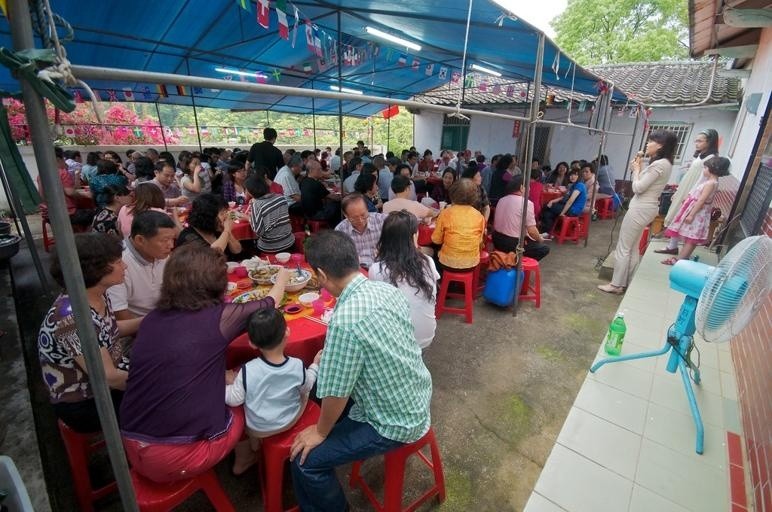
[45,0,651,141]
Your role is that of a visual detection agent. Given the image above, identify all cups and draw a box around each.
[438,200,447,210]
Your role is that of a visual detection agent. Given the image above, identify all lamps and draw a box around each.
[360,24,424,54]
[469,61,504,80]
[329,84,363,96]
[213,67,270,79]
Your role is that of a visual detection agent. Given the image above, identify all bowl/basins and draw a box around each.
[247,264,284,285]
[274,253,291,263]
[298,293,320,308]
[271,268,312,292]
[228,201,236,208]
[225,261,239,273]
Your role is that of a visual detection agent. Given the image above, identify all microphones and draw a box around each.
[631,151,644,173]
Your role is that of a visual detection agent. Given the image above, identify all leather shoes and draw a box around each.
[598,283,624,293]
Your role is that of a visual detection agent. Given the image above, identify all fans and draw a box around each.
[587,231,771,456]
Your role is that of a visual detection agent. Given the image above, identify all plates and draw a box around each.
[232,289,287,308]
[284,304,303,314]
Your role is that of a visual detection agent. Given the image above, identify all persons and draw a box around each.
[596,128,677,296]
[659,157,731,266]
[654,128,720,254]
[32,118,616,512]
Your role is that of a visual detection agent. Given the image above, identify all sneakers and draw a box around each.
[661,257,678,265]
[655,247,679,254]
[539,232,552,240]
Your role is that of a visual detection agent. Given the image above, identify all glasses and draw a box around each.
[693,139,707,144]
[348,214,371,223]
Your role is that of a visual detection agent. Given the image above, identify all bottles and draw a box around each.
[605,311,627,355]
